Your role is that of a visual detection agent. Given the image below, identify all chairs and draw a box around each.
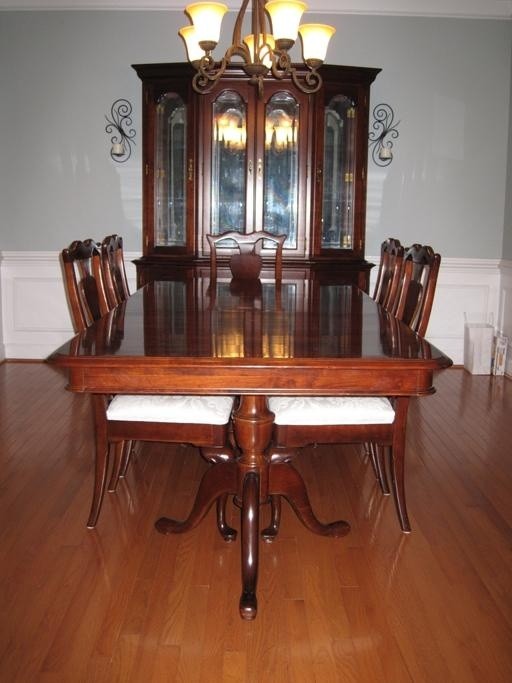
[204,231,287,284]
[60,239,238,542]
[372,236,406,320]
[100,235,133,310]
[261,243,441,541]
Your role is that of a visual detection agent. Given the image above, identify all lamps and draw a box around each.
[368,104,403,166]
[103,98,138,164]
[179,0,336,94]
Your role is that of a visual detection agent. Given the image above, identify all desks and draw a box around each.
[42,286,457,619]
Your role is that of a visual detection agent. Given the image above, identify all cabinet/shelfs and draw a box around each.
[126,60,382,295]
